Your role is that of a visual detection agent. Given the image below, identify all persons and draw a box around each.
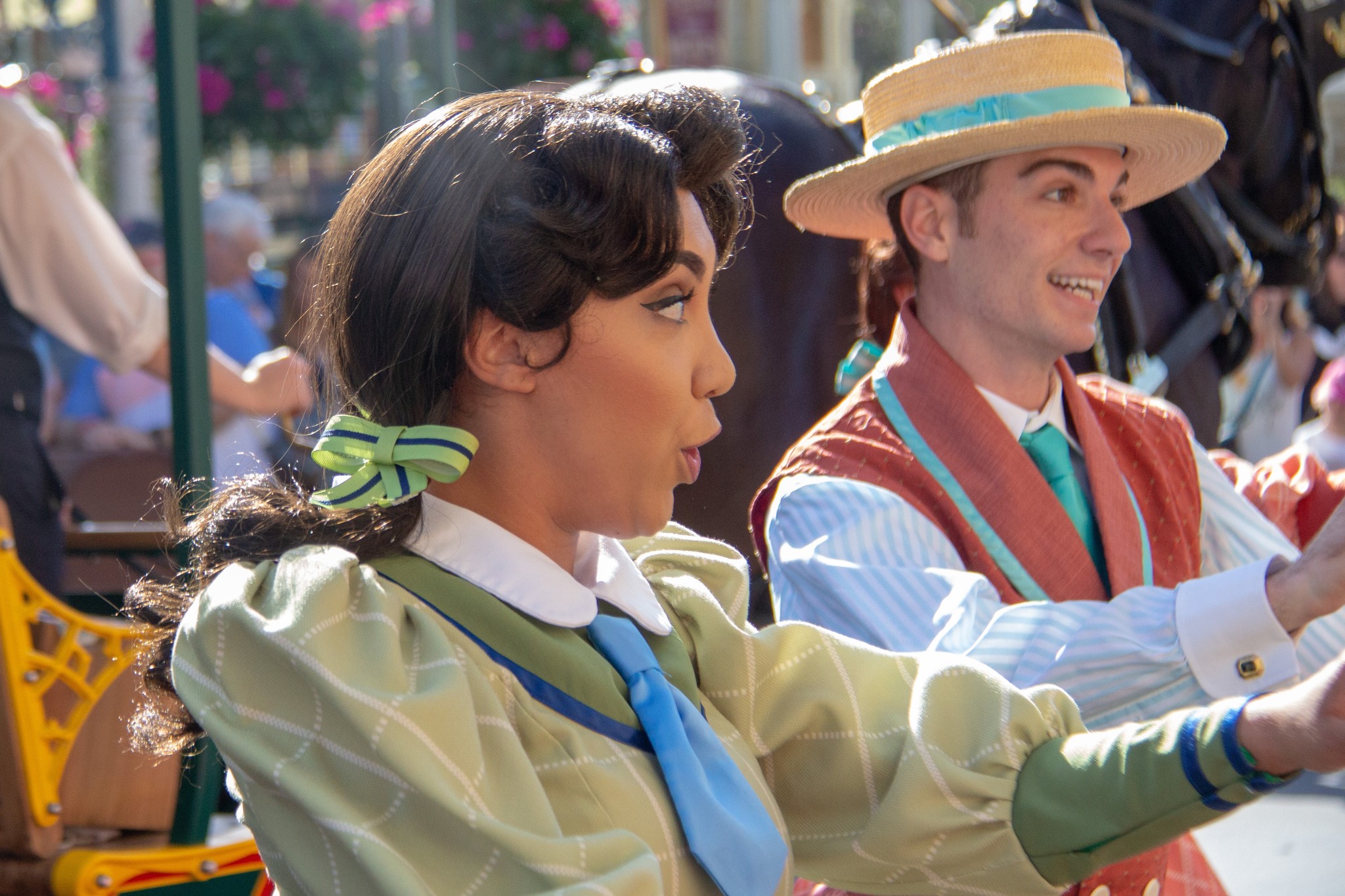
[30,194,348,497]
[749,32,1345,896]
[0,88,315,590]
[1210,63,1345,549]
[124,83,1345,896]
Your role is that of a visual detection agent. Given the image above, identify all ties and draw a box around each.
[586,615,788,896]
[1019,423,1097,572]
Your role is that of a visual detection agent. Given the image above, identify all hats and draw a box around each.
[782,29,1230,240]
[1317,355,1345,404]
